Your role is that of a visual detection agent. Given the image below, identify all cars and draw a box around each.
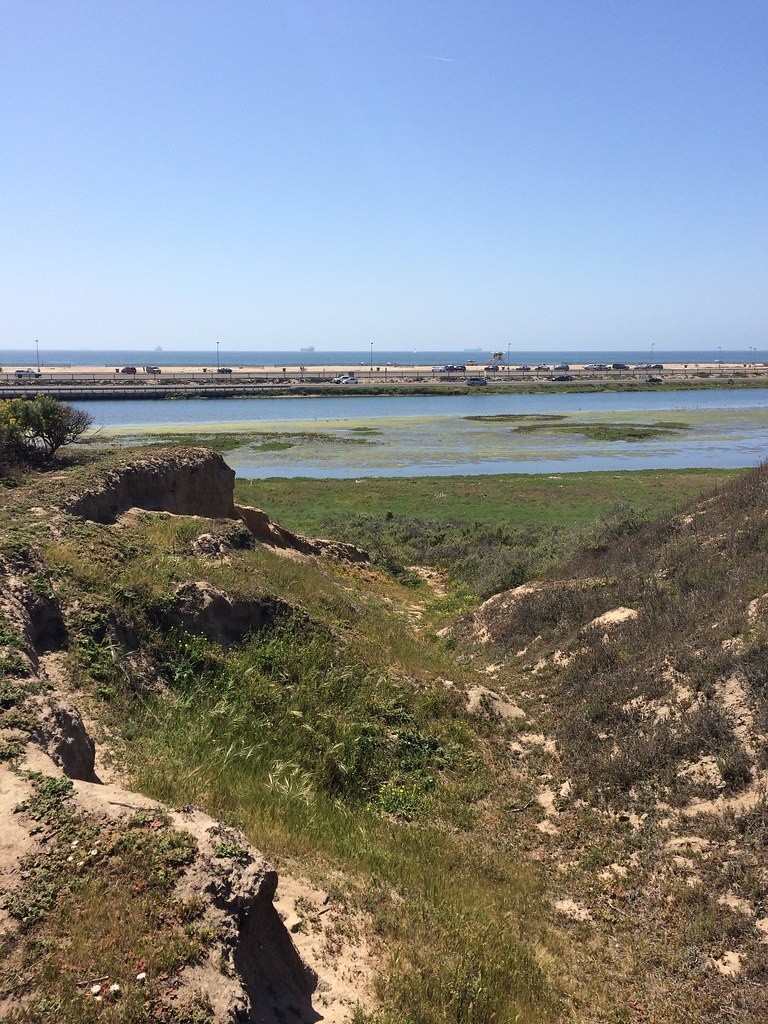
[217,367,232,374]
[145,365,162,374]
[121,366,137,374]
[342,376,358,384]
[465,377,488,387]
[443,361,664,382]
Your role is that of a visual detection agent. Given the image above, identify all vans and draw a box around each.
[14,370,40,378]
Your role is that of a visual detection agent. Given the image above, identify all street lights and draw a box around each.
[507,342,511,371]
[35,339,40,372]
[216,341,219,373]
[370,342,373,371]
[650,342,655,365]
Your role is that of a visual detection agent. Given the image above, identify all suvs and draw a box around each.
[331,376,350,384]
